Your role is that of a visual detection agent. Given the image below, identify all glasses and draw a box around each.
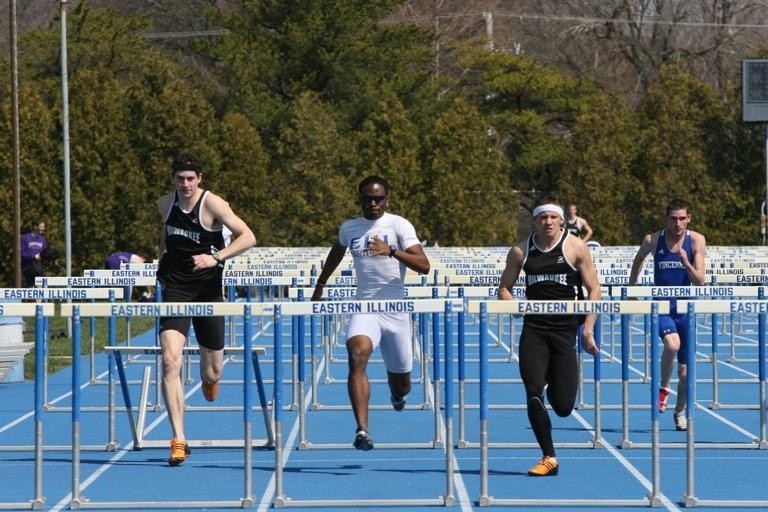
[358,193,388,204]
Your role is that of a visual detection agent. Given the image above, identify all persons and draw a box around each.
[310,177,430,451]
[156,154,257,466]
[498,196,601,475]
[105,250,151,301]
[21,219,48,303]
[560,203,593,242]
[628,200,705,431]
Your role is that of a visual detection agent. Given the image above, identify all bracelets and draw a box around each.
[315,280,326,289]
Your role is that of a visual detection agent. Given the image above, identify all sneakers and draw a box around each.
[673,408,689,432]
[200,378,220,402]
[353,429,375,452]
[167,437,192,466]
[657,384,671,414]
[390,392,409,412]
[527,454,560,477]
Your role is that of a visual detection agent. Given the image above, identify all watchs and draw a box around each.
[212,252,221,264]
[389,245,396,257]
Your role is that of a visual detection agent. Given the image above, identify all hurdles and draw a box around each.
[447,286,610,448]
[1,302,55,509]
[261,298,465,508]
[288,287,446,449]
[610,284,767,450]
[675,299,768,509]
[545,244,768,411]
[1,288,125,453]
[32,246,527,412]
[467,298,671,508]
[58,301,260,507]
[103,345,277,452]
